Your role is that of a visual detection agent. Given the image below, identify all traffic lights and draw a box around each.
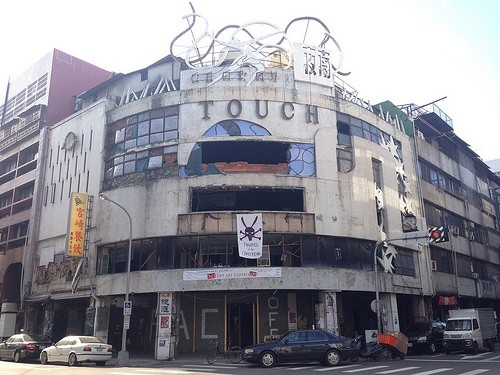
[428,227,446,243]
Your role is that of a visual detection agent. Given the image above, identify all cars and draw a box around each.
[240,329,357,369]
[0,333,52,363]
[38,335,113,367]
[400,320,446,354]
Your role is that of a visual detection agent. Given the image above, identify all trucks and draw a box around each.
[442,308,498,354]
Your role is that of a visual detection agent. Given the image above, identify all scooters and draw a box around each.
[351,334,384,363]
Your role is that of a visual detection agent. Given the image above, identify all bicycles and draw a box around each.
[207,340,245,365]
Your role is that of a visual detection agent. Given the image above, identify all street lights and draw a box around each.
[99,193,133,367]
